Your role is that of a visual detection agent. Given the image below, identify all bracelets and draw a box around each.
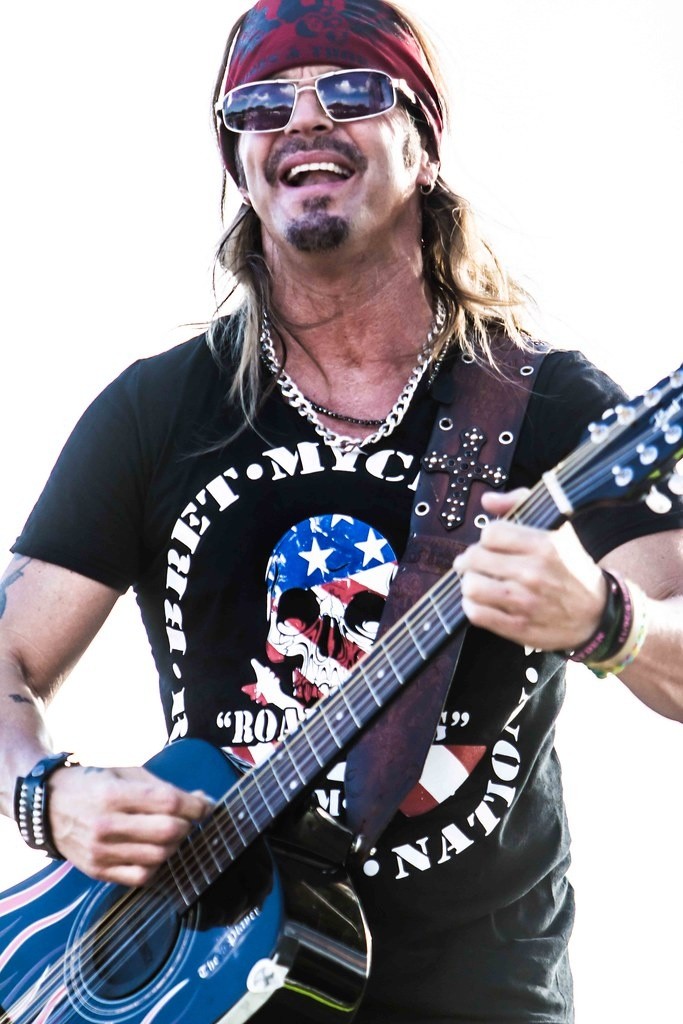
[562,565,653,681]
[11,750,81,863]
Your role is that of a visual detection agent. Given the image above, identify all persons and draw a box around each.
[1,0,682,1024]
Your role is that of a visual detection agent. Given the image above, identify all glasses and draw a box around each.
[214,69,416,134]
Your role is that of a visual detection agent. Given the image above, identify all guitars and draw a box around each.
[2,362,683,1024]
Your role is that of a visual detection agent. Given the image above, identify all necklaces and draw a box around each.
[255,295,446,456]
